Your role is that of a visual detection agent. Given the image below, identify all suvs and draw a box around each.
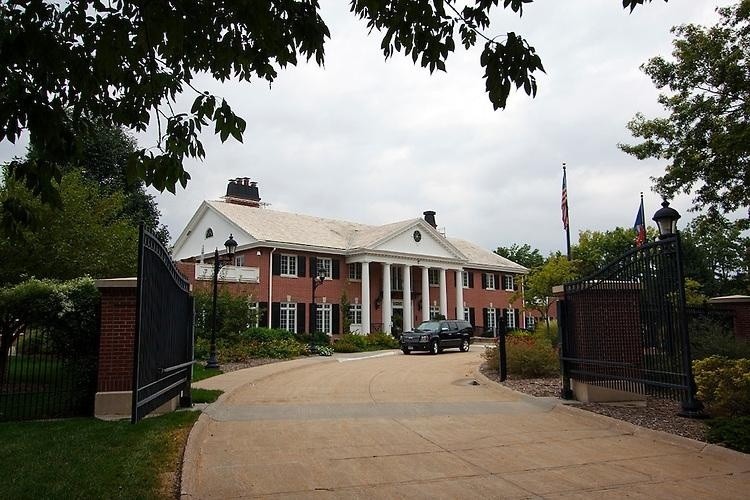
[400,320,476,356]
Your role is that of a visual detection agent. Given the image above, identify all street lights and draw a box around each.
[310,267,326,353]
[204,235,238,370]
[654,194,712,419]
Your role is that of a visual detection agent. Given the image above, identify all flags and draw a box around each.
[561,173,569,231]
[634,202,646,246]
